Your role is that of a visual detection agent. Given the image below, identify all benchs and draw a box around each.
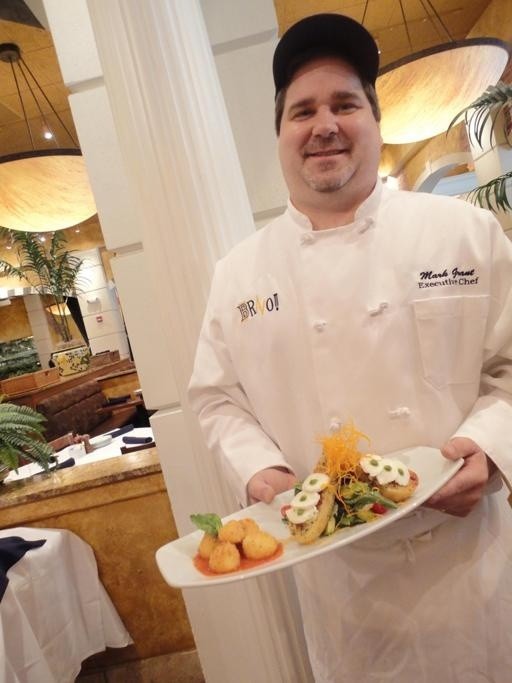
[18,379,137,438]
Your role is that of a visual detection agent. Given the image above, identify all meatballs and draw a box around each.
[198,517,277,574]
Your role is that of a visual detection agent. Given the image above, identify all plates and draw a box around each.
[154,444,467,591]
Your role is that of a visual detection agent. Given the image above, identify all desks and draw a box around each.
[1,526,113,683]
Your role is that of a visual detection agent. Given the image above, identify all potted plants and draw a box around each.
[0,224,96,377]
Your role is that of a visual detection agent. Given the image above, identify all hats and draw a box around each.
[273,12,380,102]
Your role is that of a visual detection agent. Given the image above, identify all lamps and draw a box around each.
[0,37,97,236]
[361,0,511,146]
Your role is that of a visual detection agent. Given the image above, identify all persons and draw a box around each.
[188,13,512,683]
